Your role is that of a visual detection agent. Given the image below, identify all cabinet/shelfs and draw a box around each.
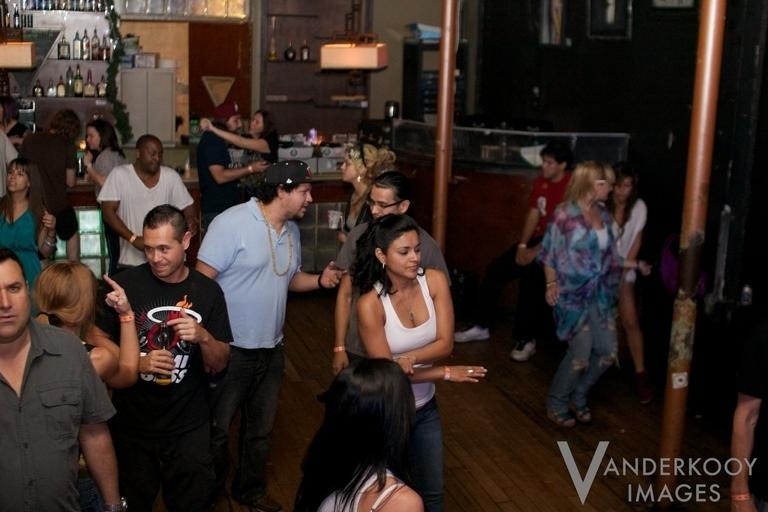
[22,7,117,103]
[264,58,317,105]
[399,34,471,121]
[391,115,631,285]
[115,68,177,152]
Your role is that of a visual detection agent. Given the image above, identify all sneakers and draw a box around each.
[453,323,651,426]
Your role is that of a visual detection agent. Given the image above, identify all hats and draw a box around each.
[212,100,239,120]
[261,161,315,184]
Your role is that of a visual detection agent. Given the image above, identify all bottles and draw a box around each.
[154,321,172,379]
[0,0,110,98]
[300,38,311,61]
[284,38,297,61]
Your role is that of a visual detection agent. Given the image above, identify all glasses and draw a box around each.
[365,198,402,208]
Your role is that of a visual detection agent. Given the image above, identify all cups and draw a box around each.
[64,170,75,187]
[383,100,400,121]
[327,210,344,230]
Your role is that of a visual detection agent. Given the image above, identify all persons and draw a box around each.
[0,99,768,512]
